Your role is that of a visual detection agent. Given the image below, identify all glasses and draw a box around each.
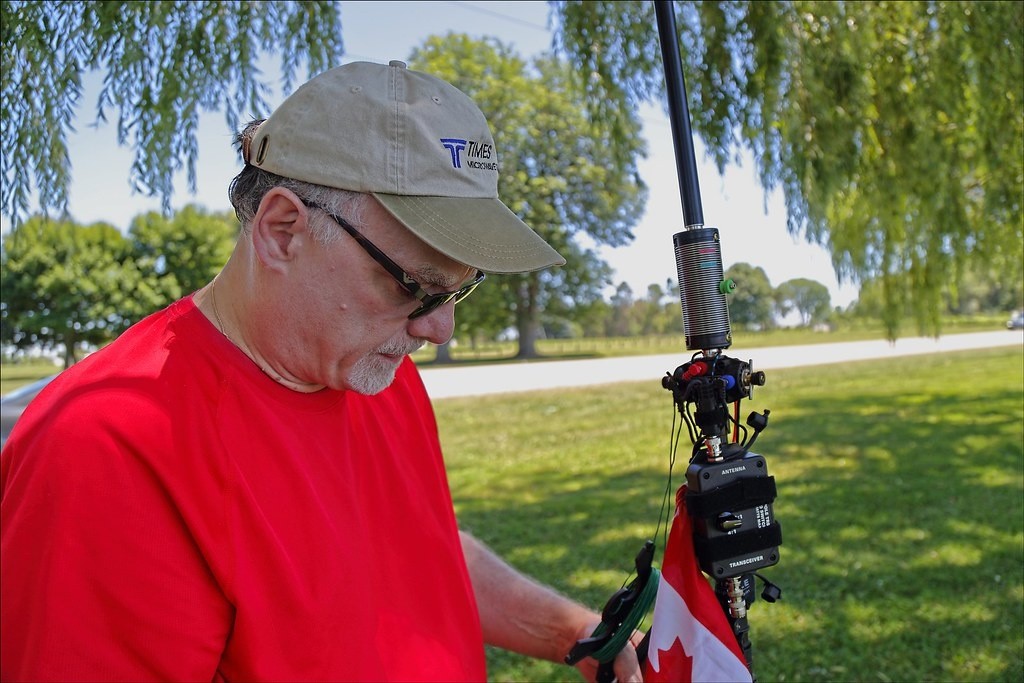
[300,194,486,319]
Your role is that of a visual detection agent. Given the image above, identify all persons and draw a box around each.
[0,61,653,683]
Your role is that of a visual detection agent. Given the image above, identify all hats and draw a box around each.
[247,60,569,275]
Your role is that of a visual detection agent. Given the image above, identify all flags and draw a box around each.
[640,485,754,683]
